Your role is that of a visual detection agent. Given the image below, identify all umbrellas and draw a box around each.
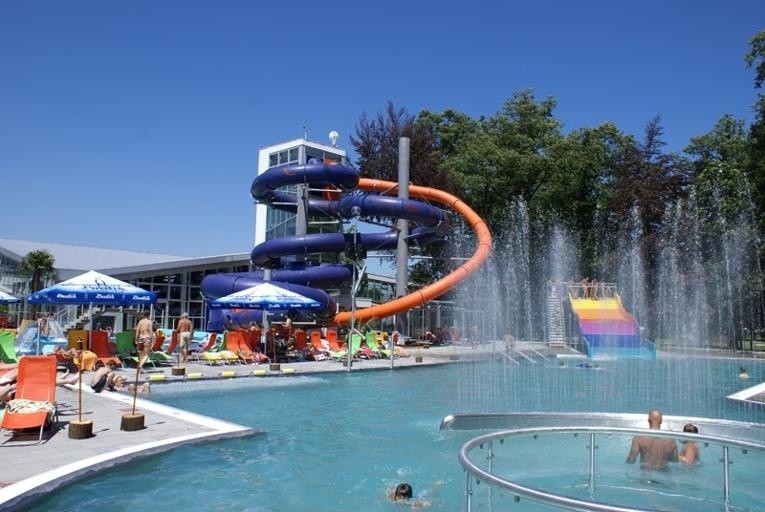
[204,281,321,354]
[24,268,160,353]
[0,288,21,306]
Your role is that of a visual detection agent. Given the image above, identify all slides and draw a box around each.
[567,293,656,360]
[199,163,493,332]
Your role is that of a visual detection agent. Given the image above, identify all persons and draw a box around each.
[212,312,480,361]
[381,478,445,510]
[133,309,154,374]
[625,408,681,473]
[0,343,152,399]
[172,311,193,364]
[548,277,615,301]
[736,364,751,379]
[672,423,703,469]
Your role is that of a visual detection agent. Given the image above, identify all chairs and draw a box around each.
[69,327,409,367]
[0,356,58,447]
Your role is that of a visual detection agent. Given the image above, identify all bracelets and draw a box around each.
[135,339,137,342]
[681,456,687,462]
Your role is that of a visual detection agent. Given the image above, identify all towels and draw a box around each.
[5,399,56,417]
